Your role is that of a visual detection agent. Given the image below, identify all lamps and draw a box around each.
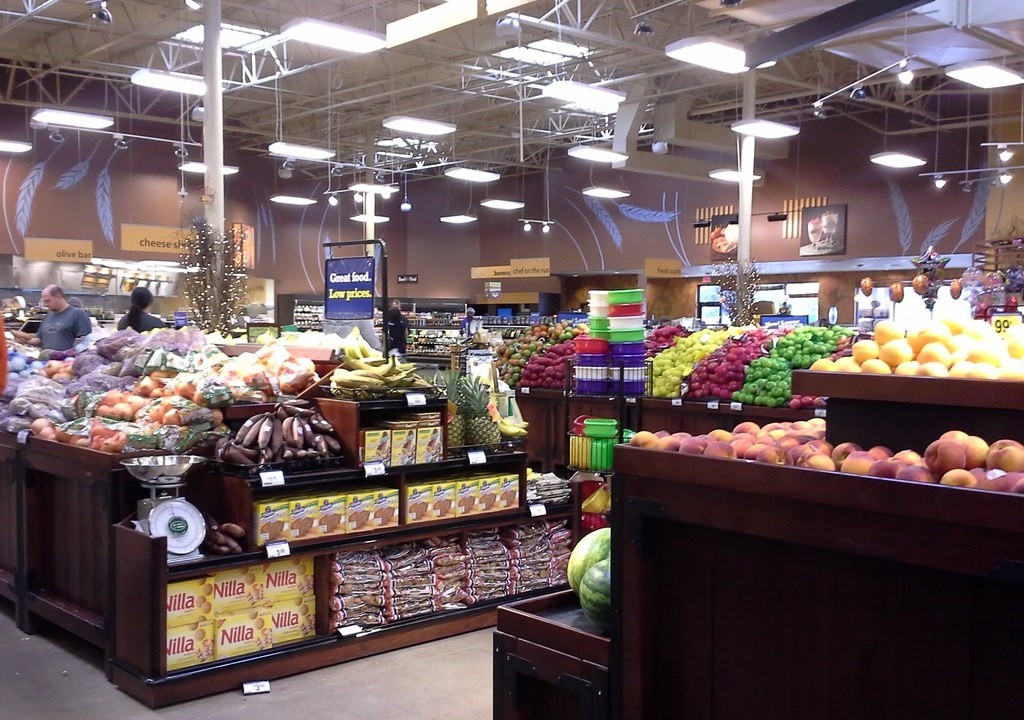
[401,173,412,211]
[47,127,64,142]
[269,60,336,160]
[92,0,112,25]
[332,165,344,176]
[375,170,385,182]
[567,138,630,162]
[542,80,627,106]
[382,115,457,135]
[111,133,128,149]
[0,139,32,152]
[131,68,206,96]
[652,98,668,155]
[444,167,501,182]
[665,35,776,74]
[31,23,115,128]
[944,56,1024,89]
[173,143,189,157]
[897,59,913,84]
[850,83,867,102]
[729,214,738,224]
[869,107,928,169]
[192,101,205,122]
[480,183,525,210]
[767,213,787,221]
[496,17,521,42]
[176,161,240,176]
[280,0,385,54]
[582,162,631,198]
[919,88,1024,194]
[278,156,295,178]
[812,100,828,119]
[633,15,654,37]
[693,220,710,227]
[729,119,800,139]
[709,147,761,182]
[348,173,399,194]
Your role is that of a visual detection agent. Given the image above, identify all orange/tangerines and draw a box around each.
[31,350,315,452]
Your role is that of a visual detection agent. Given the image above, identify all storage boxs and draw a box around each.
[167,557,316,672]
[406,472,519,523]
[360,426,443,467]
[252,485,399,546]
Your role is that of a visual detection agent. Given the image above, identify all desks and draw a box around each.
[514,387,827,469]
[1,428,220,651]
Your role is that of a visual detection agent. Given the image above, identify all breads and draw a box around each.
[329,518,573,630]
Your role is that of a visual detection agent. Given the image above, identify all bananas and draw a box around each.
[497,418,529,436]
[257,327,276,344]
[205,517,243,555]
[330,327,421,397]
[218,399,341,472]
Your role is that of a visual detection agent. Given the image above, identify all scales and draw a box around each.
[120,455,207,565]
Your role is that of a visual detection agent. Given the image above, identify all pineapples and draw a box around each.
[436,370,501,454]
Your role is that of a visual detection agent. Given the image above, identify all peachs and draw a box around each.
[628,419,1024,494]
[810,317,1024,383]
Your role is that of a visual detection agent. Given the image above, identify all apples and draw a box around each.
[497,322,589,389]
[647,325,851,408]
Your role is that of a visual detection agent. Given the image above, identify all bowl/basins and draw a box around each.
[574,288,647,395]
[569,415,633,530]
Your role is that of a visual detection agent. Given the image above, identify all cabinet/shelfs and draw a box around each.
[294,299,529,370]
[106,360,582,708]
[492,371,1023,720]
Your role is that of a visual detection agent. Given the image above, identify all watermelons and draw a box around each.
[567,527,612,634]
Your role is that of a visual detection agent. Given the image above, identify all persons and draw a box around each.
[388,300,400,320]
[389,316,409,358]
[19,284,92,351]
[460,307,475,336]
[118,287,165,334]
